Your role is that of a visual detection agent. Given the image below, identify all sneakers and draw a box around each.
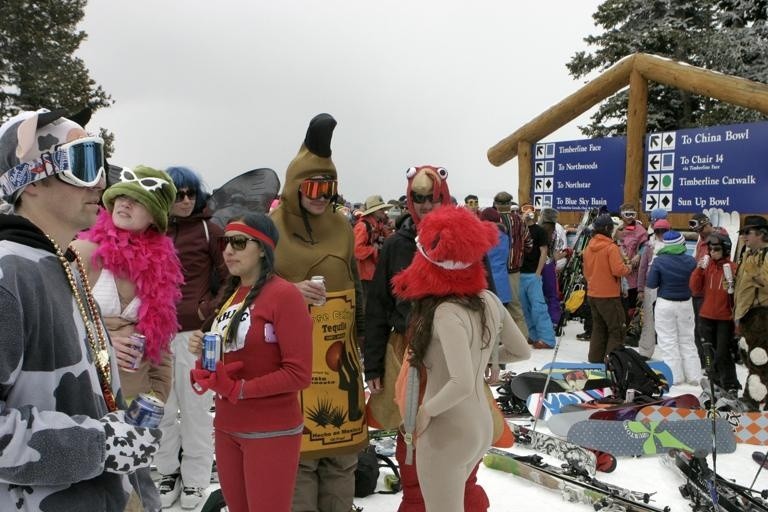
[157,473,180,507]
[576,331,591,340]
[534,340,550,349]
[180,487,202,508]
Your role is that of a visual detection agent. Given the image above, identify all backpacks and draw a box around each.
[605,344,664,399]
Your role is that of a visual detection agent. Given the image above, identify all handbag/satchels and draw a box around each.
[356,444,380,497]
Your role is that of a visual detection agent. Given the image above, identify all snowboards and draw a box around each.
[495,359,768,458]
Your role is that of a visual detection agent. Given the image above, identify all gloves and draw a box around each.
[99,410,161,475]
[189,358,245,400]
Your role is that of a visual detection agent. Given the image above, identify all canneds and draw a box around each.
[120,333,145,373]
[124,393,165,428]
[311,276,326,307]
[202,332,223,371]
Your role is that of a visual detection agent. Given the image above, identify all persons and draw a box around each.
[345,194,402,355]
[60,163,182,415]
[187,213,313,512]
[465,193,567,348]
[576,205,712,386]
[268,112,369,510]
[1,104,164,512]
[154,168,231,509]
[689,216,768,412]
[385,206,532,512]
[360,163,496,511]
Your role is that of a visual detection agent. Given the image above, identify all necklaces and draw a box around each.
[43,232,115,388]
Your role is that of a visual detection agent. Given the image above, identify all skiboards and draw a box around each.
[482,420,768,512]
[366,428,397,460]
[553,207,597,336]
